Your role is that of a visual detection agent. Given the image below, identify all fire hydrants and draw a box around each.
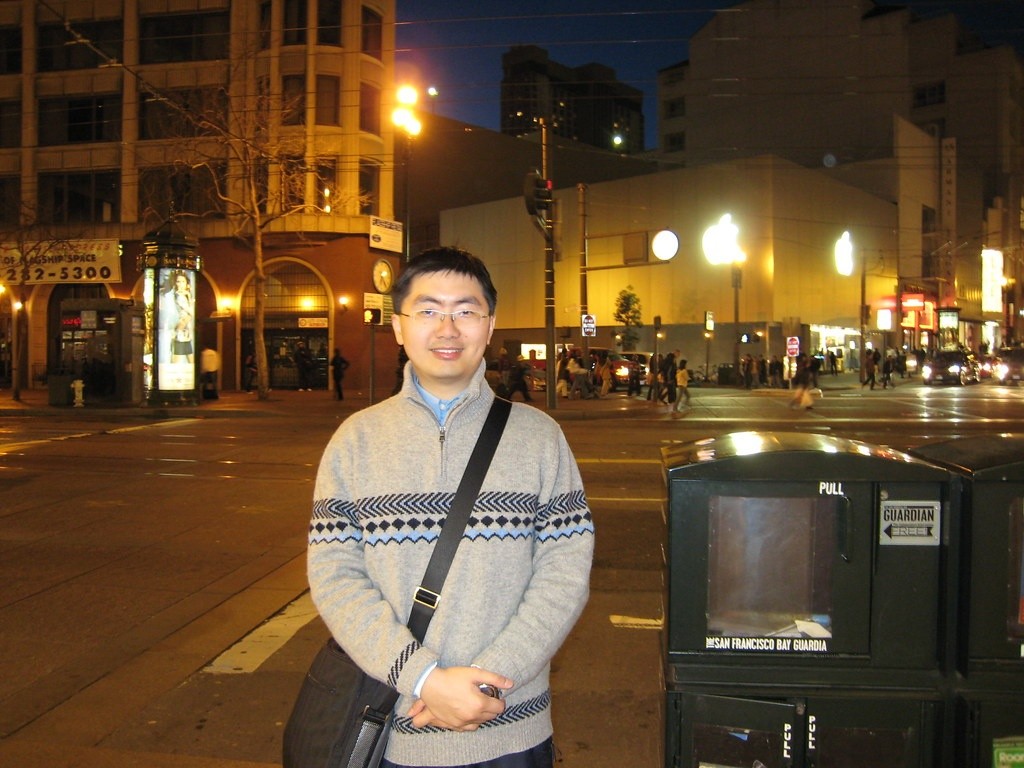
[69,379,86,407]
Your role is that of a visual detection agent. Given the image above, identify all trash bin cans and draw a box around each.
[661,432,1024,768]
[718,363,733,384]
[47,372,74,408]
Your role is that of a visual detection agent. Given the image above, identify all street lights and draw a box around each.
[704,333,711,382]
[390,83,425,394]
[654,333,664,402]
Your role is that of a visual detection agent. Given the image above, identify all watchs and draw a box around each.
[479,684,499,700]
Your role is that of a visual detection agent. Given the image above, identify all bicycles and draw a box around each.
[692,365,718,384]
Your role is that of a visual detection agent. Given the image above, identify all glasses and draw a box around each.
[400,309,489,329]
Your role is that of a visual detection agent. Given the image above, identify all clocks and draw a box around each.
[373,259,394,295]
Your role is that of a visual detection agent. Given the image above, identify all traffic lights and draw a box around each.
[741,333,749,344]
[364,308,381,323]
[523,172,553,215]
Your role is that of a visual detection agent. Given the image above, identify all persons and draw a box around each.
[247,353,260,394]
[306,246,594,768]
[918,347,926,367]
[627,354,642,396]
[555,349,614,399]
[740,353,789,390]
[294,342,312,391]
[862,347,907,391]
[330,347,349,400]
[159,270,194,363]
[499,347,514,386]
[646,353,692,415]
[505,354,535,401]
[199,342,219,400]
[792,352,821,387]
[829,352,838,377]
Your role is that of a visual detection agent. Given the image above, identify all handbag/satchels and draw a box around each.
[282,637,398,768]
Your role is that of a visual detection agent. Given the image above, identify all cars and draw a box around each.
[922,351,992,386]
[991,348,1024,386]
[485,347,654,392]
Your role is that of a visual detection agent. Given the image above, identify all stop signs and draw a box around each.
[582,315,597,337]
[787,336,800,357]
